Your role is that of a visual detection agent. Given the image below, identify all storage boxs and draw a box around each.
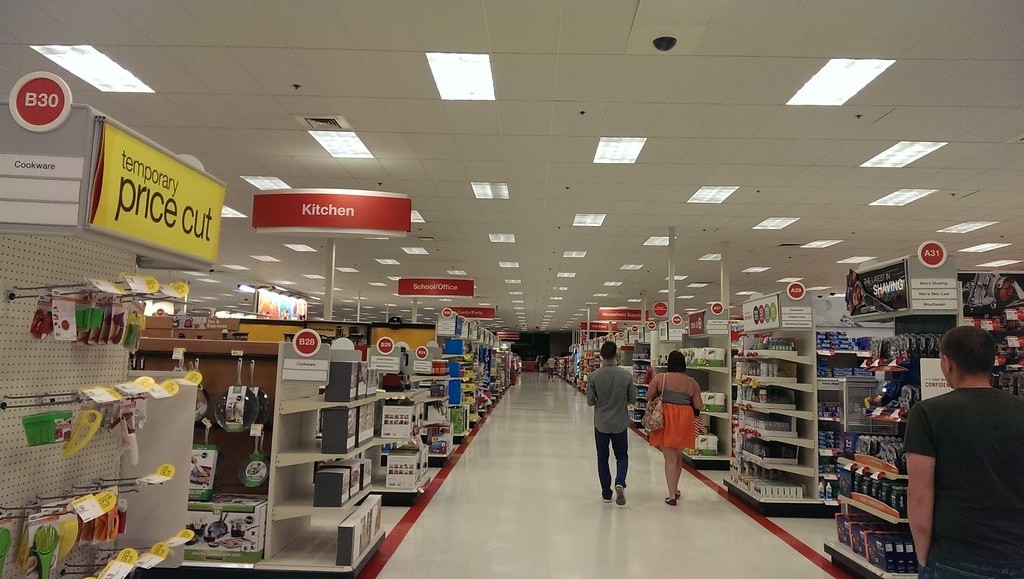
[835,513,920,573]
[449,362,460,376]
[384,404,429,488]
[314,361,382,565]
[451,406,470,433]
[450,380,461,404]
[445,339,464,354]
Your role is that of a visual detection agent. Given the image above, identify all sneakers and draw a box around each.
[674,490,681,499]
[601,493,612,503]
[665,497,677,505]
[616,485,627,505]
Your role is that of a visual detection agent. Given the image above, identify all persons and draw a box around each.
[535,355,543,371]
[587,341,636,506]
[646,351,704,505]
[547,356,555,382]
[902,326,1024,579]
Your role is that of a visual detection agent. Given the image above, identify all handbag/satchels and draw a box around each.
[641,392,666,432]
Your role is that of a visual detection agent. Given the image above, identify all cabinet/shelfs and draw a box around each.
[129,338,385,578]
[824,378,925,579]
[408,347,455,466]
[569,322,737,470]
[722,297,908,517]
[357,345,431,503]
[442,355,473,444]
